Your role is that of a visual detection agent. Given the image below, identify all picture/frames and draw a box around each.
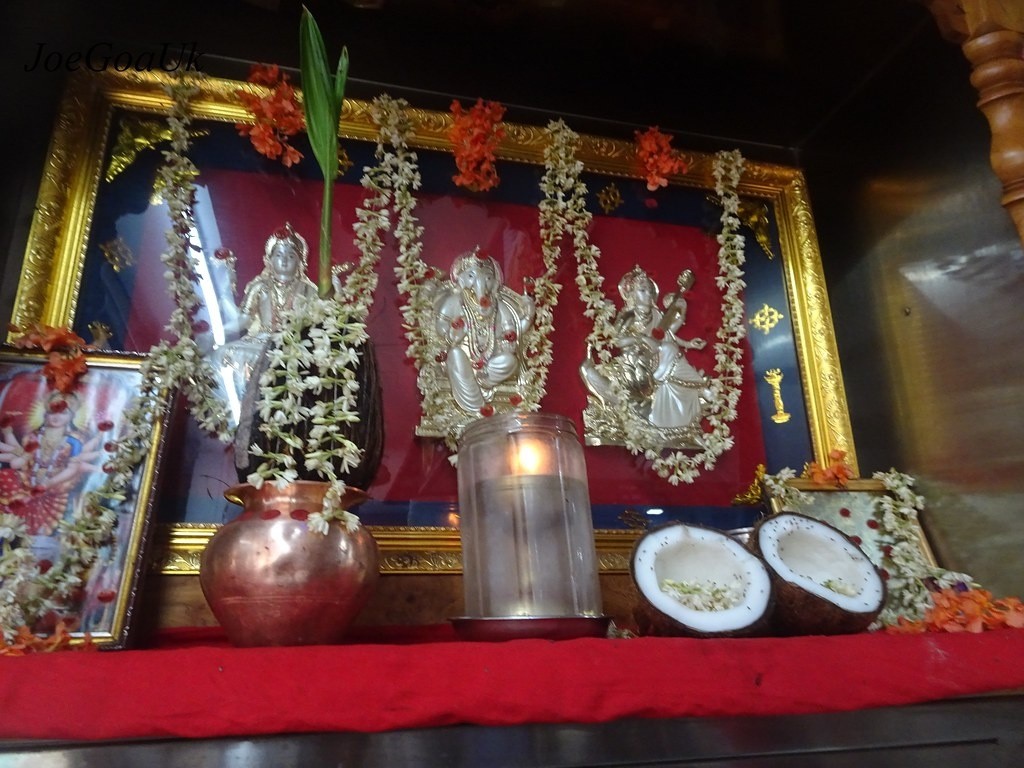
[0,346,181,650]
[8,42,860,575]
[762,475,940,630]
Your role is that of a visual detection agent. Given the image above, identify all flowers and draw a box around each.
[0,54,1024,656]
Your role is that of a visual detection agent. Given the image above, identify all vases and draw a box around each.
[199,481,378,647]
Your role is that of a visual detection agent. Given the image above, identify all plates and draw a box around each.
[449,616,618,639]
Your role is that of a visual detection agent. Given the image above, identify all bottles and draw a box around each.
[458,414,600,618]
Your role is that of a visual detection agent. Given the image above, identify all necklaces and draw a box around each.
[635,306,653,326]
[272,280,297,295]
[463,298,497,361]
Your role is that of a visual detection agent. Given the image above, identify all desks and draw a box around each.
[0,628,1024,768]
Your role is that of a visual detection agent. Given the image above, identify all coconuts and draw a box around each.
[745,510,887,633]
[629,520,780,638]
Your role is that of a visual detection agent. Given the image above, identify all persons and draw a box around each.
[578,268,709,428]
[204,228,341,434]
[434,250,536,412]
